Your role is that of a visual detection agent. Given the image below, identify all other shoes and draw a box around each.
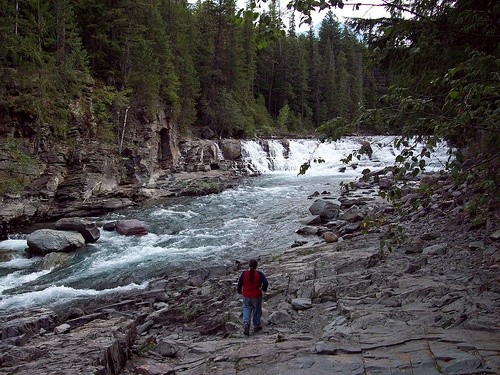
[254,326,262,332]
[243,325,250,335]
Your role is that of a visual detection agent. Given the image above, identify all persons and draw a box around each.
[237,259,269,335]
